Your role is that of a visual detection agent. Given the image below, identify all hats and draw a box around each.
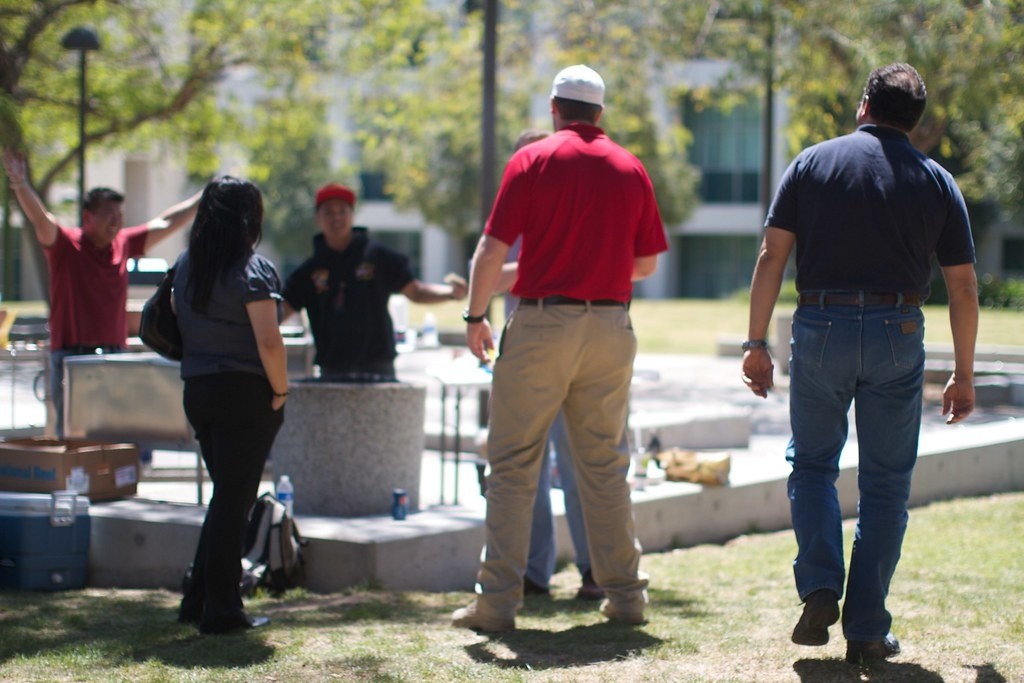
[552,64,605,105]
[315,183,355,209]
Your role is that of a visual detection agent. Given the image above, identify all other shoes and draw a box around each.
[582,572,603,598]
[453,605,485,631]
[600,598,649,625]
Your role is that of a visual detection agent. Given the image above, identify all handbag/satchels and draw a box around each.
[138,263,183,361]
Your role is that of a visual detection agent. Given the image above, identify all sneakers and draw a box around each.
[791,589,840,646]
[846,633,900,665]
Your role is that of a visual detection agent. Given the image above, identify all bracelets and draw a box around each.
[741,339,766,351]
[8,178,27,190]
[273,391,289,397]
[463,313,486,323]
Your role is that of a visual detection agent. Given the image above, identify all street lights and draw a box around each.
[461,1,498,234]
[57,23,103,229]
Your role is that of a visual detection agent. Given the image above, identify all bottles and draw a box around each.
[277,475,294,518]
[422,313,439,347]
[633,446,648,492]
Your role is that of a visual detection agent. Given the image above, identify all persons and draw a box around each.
[0,146,205,444]
[277,181,468,384]
[741,61,979,661]
[140,173,289,638]
[448,63,671,634]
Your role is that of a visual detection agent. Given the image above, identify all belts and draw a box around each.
[519,294,628,309]
[797,292,923,308]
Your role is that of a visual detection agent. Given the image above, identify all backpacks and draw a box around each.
[239,491,305,593]
